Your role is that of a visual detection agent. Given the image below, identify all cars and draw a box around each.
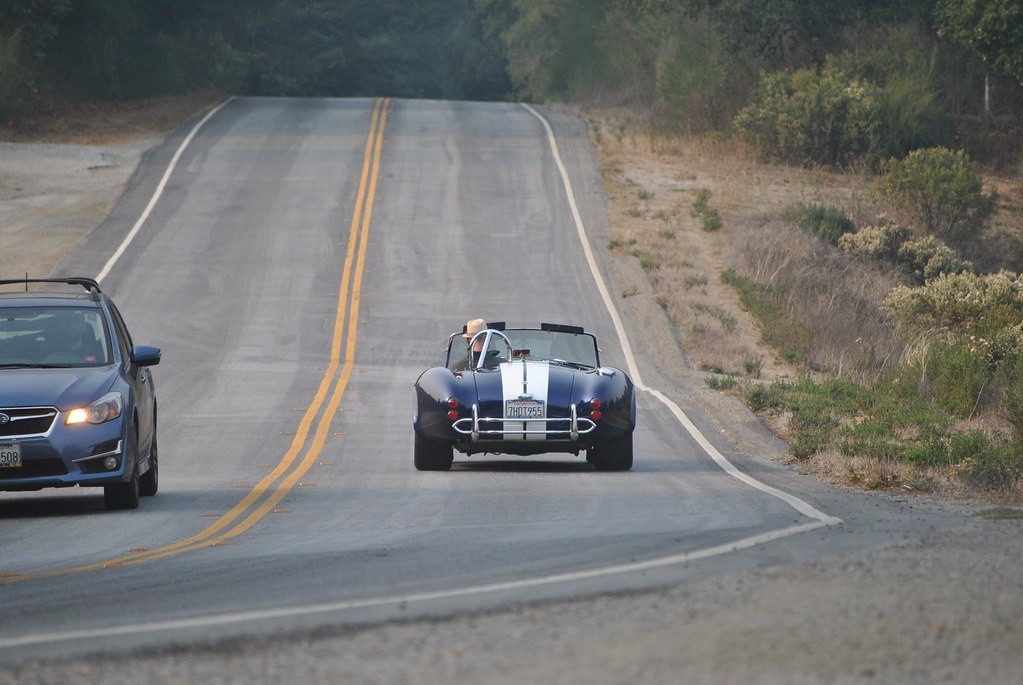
[0,278,162,510]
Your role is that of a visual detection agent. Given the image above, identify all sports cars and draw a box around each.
[414,320,636,472]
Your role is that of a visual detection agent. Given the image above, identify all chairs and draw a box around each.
[41,321,96,360]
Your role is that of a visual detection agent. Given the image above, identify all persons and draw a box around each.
[58,313,102,362]
[450,318,508,379]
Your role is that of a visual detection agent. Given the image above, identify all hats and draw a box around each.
[462,318,488,338]
